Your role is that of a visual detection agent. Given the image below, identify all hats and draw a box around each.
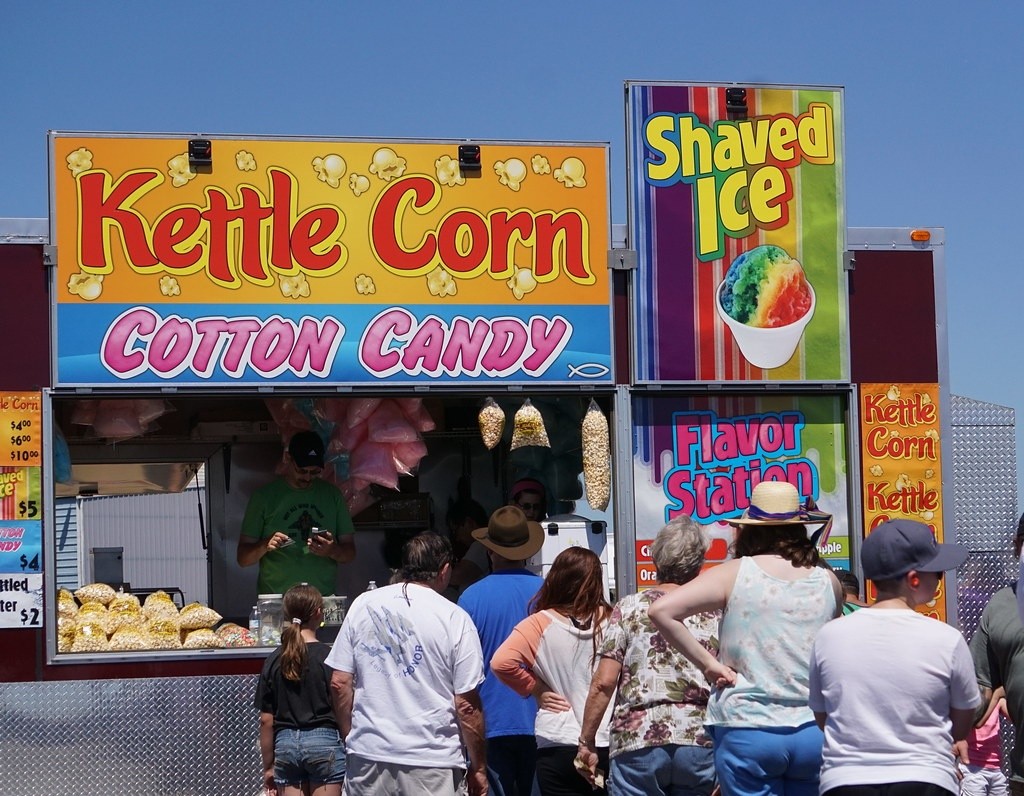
[471,505,545,560]
[862,519,966,581]
[724,481,833,524]
[289,430,326,468]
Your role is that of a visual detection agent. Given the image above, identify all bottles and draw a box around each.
[257,594,284,646]
[248,606,259,638]
[367,580,376,591]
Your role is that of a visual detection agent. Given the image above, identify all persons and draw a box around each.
[489,545,621,796]
[456,506,546,796]
[323,528,489,796]
[957,698,1013,796]
[235,428,357,598]
[452,477,547,583]
[968,511,1024,796]
[252,583,345,796]
[574,513,722,796]
[646,481,844,796]
[808,518,982,796]
[833,569,870,618]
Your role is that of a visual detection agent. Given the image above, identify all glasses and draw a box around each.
[289,459,320,475]
[515,502,545,509]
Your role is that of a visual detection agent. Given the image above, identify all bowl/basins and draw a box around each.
[715,278,816,370]
